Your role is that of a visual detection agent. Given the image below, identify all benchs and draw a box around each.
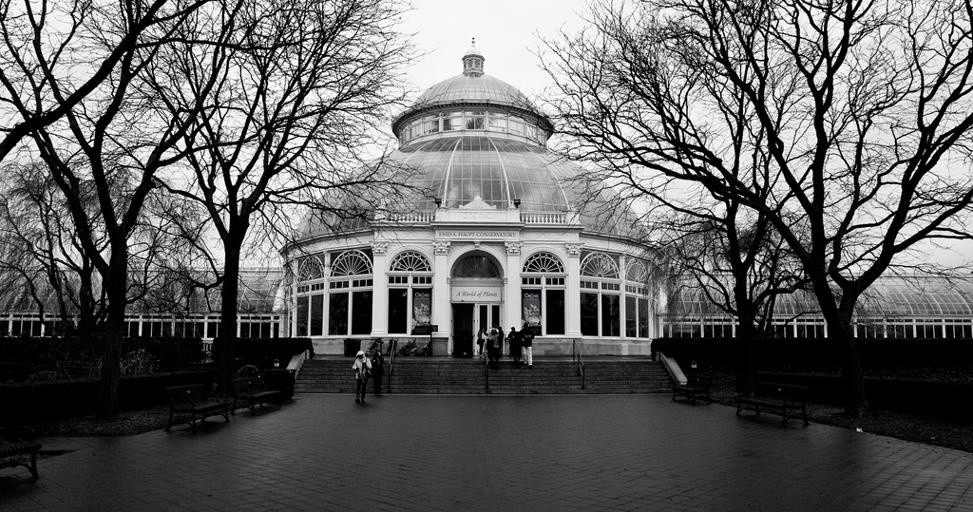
[230,374,283,417]
[0,427,42,482]
[670,375,714,404]
[165,384,229,436]
[734,382,811,427]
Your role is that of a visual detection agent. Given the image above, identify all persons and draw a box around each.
[370,351,383,396]
[476,322,534,369]
[351,350,372,405]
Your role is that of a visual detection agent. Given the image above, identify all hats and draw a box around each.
[355,350,364,357]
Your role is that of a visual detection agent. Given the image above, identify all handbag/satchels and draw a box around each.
[361,364,372,379]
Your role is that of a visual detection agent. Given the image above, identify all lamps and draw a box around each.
[433,197,441,208]
[513,198,520,209]
